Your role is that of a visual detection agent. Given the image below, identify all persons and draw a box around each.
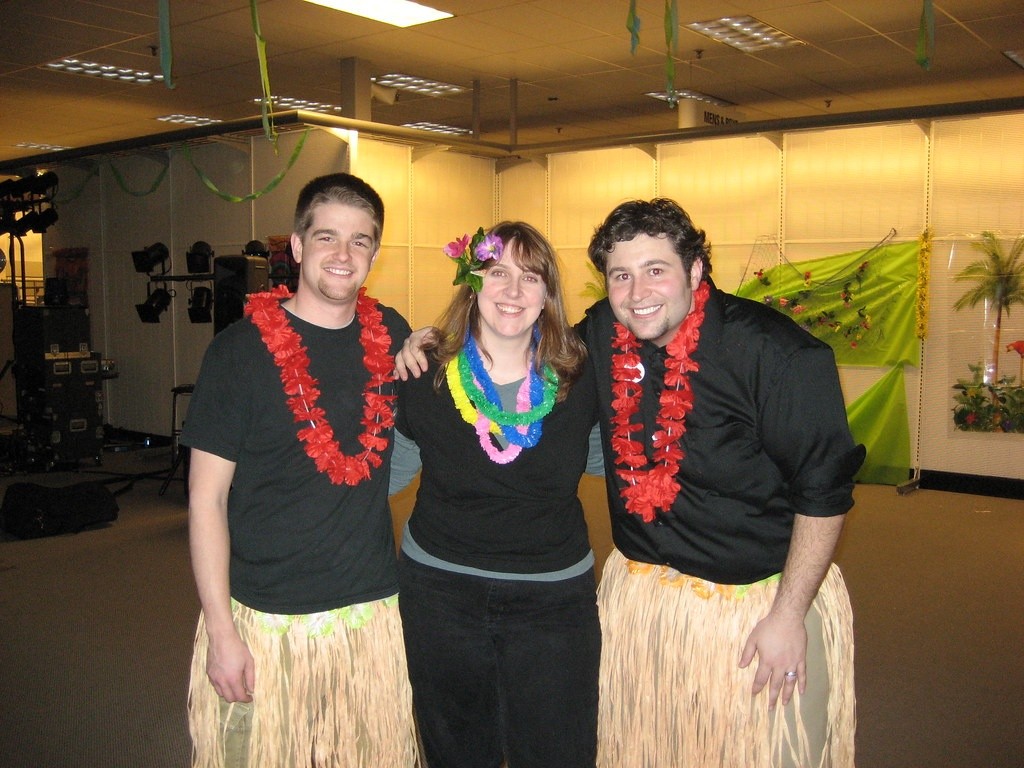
[394,197,868,768]
[176,171,413,768]
[388,222,605,768]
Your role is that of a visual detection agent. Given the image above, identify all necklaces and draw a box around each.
[445,319,558,464]
[243,285,398,486]
[610,279,711,523]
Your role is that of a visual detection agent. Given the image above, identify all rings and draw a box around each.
[403,338,410,344]
[786,672,796,676]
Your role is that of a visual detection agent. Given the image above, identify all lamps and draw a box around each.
[0,171,60,237]
[132,241,270,323]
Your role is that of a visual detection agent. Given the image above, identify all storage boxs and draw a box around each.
[12,305,104,473]
